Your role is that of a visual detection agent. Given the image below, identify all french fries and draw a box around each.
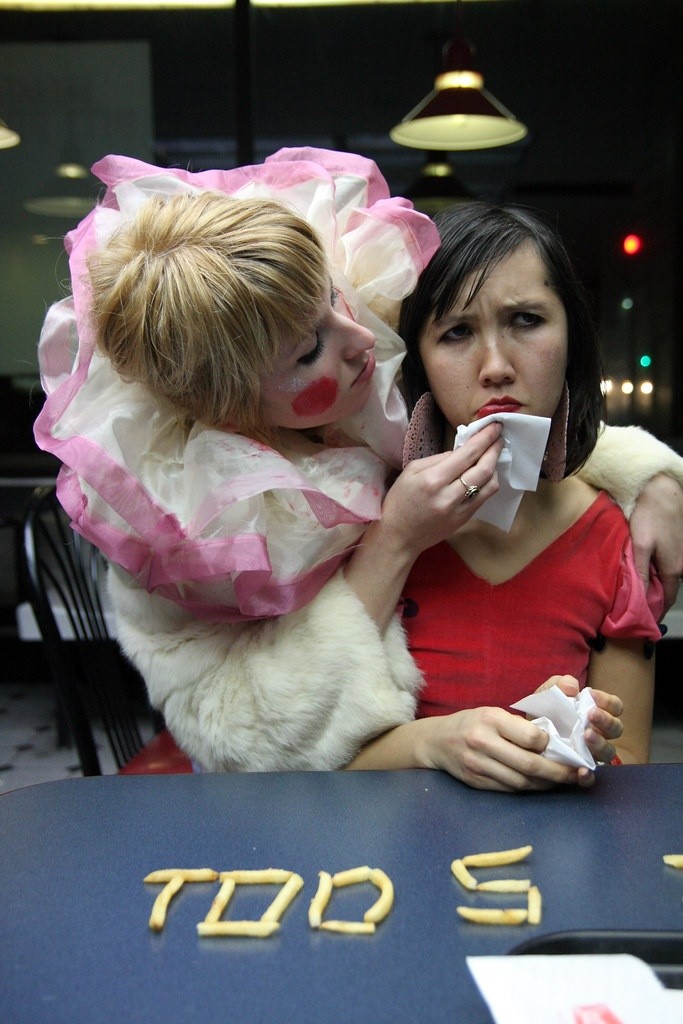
[140,844,541,936]
[663,854,683,868]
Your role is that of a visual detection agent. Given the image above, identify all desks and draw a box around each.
[1,762,683,1024]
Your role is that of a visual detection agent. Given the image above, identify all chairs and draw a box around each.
[20,481,196,775]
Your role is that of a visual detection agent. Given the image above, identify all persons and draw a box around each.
[33,147,683,773]
[349,201,658,790]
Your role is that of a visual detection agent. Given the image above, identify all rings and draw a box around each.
[459,476,479,499]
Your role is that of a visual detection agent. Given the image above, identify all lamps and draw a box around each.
[389,0,527,154]
